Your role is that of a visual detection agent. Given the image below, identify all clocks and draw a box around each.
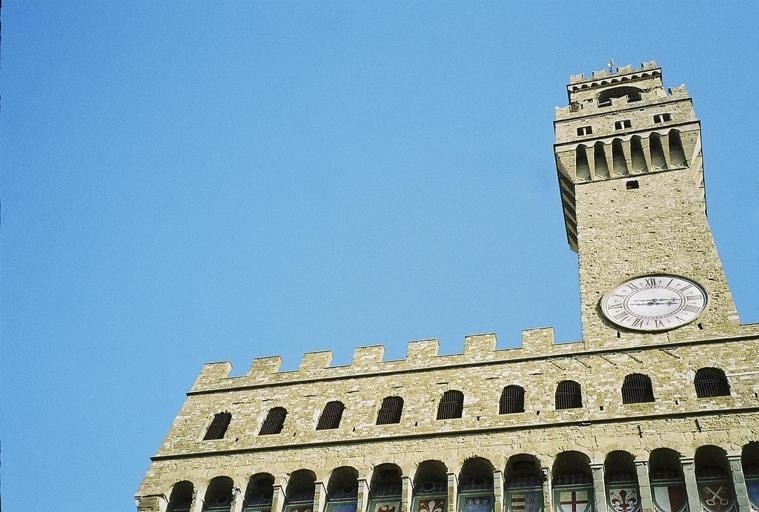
[599,273,708,334]
